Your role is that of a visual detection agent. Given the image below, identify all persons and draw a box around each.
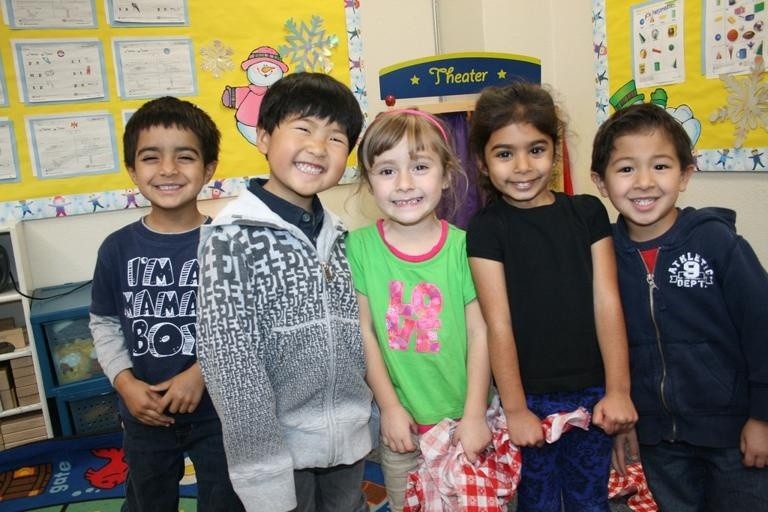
[590,103,767,511]
[464,81,638,512]
[346,107,493,512]
[88,95,244,512]
[197,72,382,512]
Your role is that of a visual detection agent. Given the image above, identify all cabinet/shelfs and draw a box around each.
[0,221,59,452]
